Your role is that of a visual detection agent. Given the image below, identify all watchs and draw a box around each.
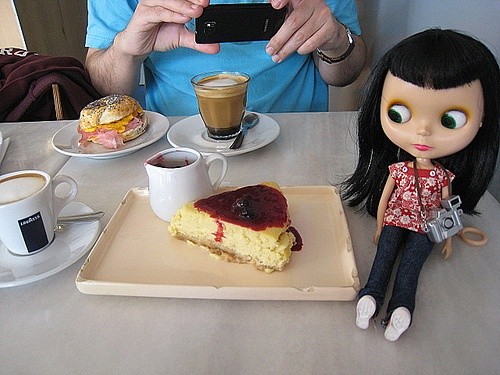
[318,23,355,65]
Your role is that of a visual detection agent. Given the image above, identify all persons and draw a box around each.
[330,27,500,340]
[84,0,367,116]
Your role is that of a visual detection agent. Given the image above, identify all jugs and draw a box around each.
[143,147,231,223]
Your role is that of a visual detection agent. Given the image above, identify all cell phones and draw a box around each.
[194,2,288,45]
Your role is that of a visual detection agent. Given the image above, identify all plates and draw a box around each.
[50,109,171,160]
[167,110,281,156]
[0,199,100,288]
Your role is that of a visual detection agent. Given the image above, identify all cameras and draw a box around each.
[422,192,465,243]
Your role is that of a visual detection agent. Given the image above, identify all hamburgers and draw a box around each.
[76,94,149,149]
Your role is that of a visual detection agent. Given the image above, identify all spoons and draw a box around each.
[229,113,260,148]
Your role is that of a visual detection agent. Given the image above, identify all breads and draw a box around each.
[169,182,292,273]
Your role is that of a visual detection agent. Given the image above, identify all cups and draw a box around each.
[191,71,251,140]
[0,169,80,255]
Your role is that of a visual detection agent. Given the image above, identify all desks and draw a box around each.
[0,111,500,375]
